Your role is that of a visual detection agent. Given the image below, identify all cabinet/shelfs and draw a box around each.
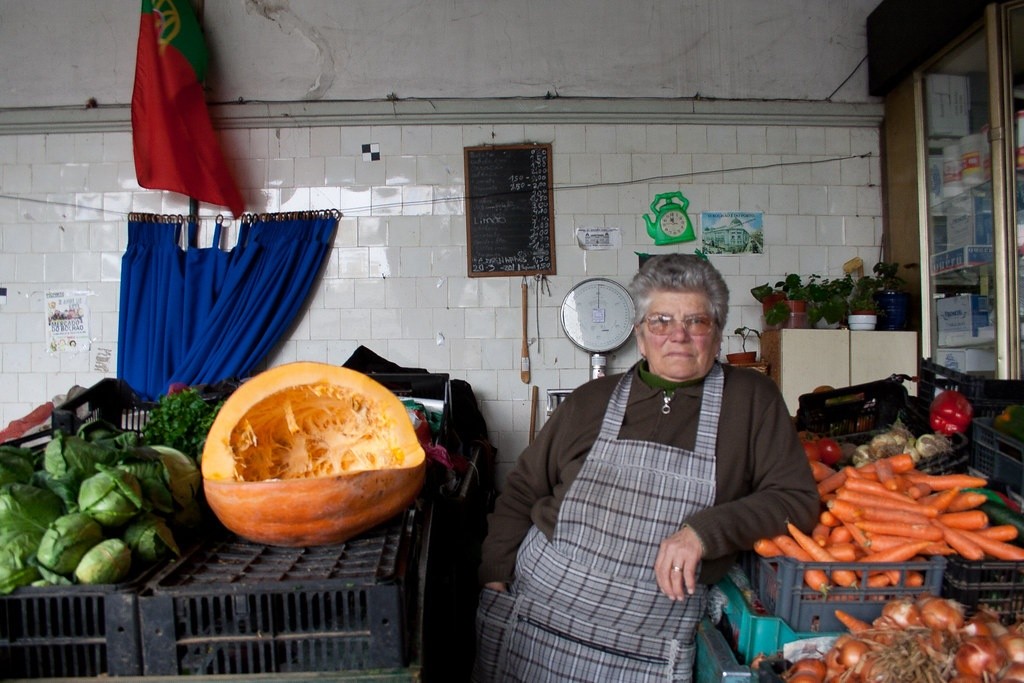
[921,0,1024,379]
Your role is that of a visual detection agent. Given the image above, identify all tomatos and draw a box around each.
[798,431,843,465]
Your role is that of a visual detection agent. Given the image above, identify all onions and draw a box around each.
[845,424,952,467]
[780,594,1024,683]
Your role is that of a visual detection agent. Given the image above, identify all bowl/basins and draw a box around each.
[848,315,876,330]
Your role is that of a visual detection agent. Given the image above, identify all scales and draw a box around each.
[546,275,636,413]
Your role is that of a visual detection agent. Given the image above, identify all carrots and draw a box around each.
[752,454,1024,600]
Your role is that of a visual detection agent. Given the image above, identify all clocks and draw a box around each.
[643,193,696,246]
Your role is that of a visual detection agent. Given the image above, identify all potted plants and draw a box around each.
[726,327,760,364]
[752,262,908,331]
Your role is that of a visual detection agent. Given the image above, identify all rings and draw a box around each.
[673,567,683,572]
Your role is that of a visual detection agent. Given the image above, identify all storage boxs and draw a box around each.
[0,551,165,678]
[970,417,1024,499]
[733,542,947,631]
[926,75,970,136]
[139,522,409,680]
[710,562,852,666]
[849,331,917,397]
[761,329,849,417]
[52,378,162,446]
[832,427,969,477]
[0,427,52,472]
[936,295,989,340]
[936,349,994,379]
[942,553,1024,625]
[930,245,993,276]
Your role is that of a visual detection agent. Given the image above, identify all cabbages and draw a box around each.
[0,419,202,595]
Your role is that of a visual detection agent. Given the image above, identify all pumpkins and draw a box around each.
[200,361,426,547]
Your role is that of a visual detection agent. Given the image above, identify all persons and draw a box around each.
[49,303,83,321]
[474,253,820,683]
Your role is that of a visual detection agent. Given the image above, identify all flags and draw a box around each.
[131,0,245,218]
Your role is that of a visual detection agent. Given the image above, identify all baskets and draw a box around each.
[732,362,768,376]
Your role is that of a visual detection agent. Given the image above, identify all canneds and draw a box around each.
[941,108,1024,198]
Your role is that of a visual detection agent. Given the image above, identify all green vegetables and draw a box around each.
[139,385,228,460]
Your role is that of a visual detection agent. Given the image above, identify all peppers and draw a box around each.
[929,389,972,436]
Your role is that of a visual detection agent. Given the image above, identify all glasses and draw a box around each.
[641,312,713,337]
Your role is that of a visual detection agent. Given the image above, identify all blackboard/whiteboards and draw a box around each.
[463,141,557,279]
